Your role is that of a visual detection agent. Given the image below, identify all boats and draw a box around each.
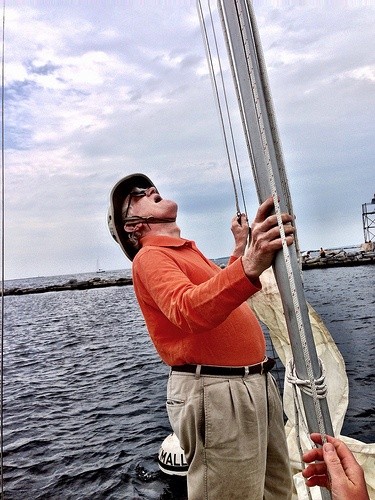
[96,269,105,273]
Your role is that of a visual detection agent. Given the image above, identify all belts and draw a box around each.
[172,357,276,376]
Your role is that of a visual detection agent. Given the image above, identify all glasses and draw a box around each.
[125,189,147,217]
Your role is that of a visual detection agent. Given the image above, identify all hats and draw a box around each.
[107,173,159,261]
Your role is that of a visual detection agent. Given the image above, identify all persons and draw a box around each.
[301,429,370,500]
[109,173,299,500]
